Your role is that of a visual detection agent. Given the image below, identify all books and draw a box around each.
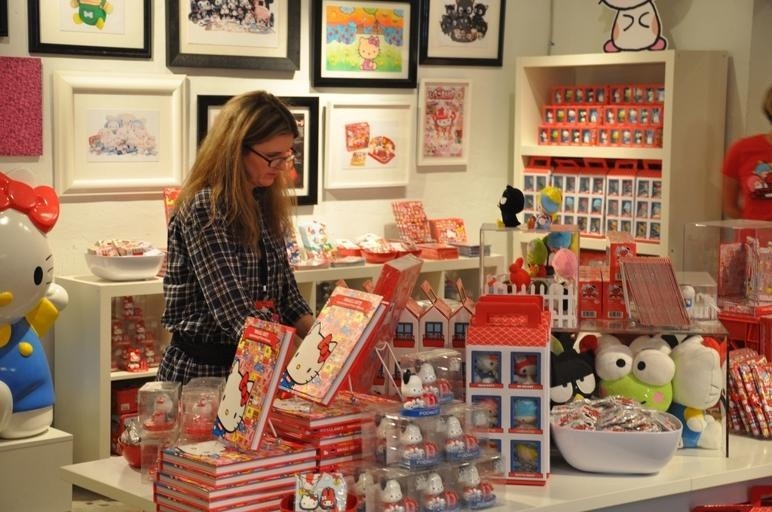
[392,200,491,260]
[278,286,390,408]
[728,349,772,440]
[283,220,335,267]
[717,239,772,315]
[268,392,376,476]
[153,435,319,512]
[621,256,690,327]
[211,318,296,453]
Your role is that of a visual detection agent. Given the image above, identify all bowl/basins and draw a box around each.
[337,243,360,263]
[551,407,683,476]
[361,242,398,264]
[85,249,165,280]
[394,244,423,258]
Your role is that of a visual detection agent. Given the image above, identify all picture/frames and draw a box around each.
[166,0,302,70]
[27,0,153,58]
[324,102,409,189]
[419,0,507,66]
[312,1,419,86]
[417,78,471,166]
[51,71,191,200]
[196,95,319,205]
[0,1,9,37]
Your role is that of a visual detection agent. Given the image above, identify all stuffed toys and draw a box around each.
[553,248,577,285]
[545,231,571,274]
[528,188,563,230]
[508,257,531,291]
[527,238,549,278]
[497,185,524,227]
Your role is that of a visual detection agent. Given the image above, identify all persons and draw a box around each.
[722,85,772,248]
[158,90,315,383]
[748,162,772,199]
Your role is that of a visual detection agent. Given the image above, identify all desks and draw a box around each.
[62,431,772,512]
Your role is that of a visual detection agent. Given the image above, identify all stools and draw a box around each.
[0,428,74,512]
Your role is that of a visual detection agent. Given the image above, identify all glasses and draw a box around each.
[246,145,296,169]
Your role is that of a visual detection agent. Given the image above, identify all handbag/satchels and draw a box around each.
[166,332,239,366]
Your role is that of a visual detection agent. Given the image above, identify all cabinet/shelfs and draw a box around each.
[55,256,507,462]
[514,50,728,276]
